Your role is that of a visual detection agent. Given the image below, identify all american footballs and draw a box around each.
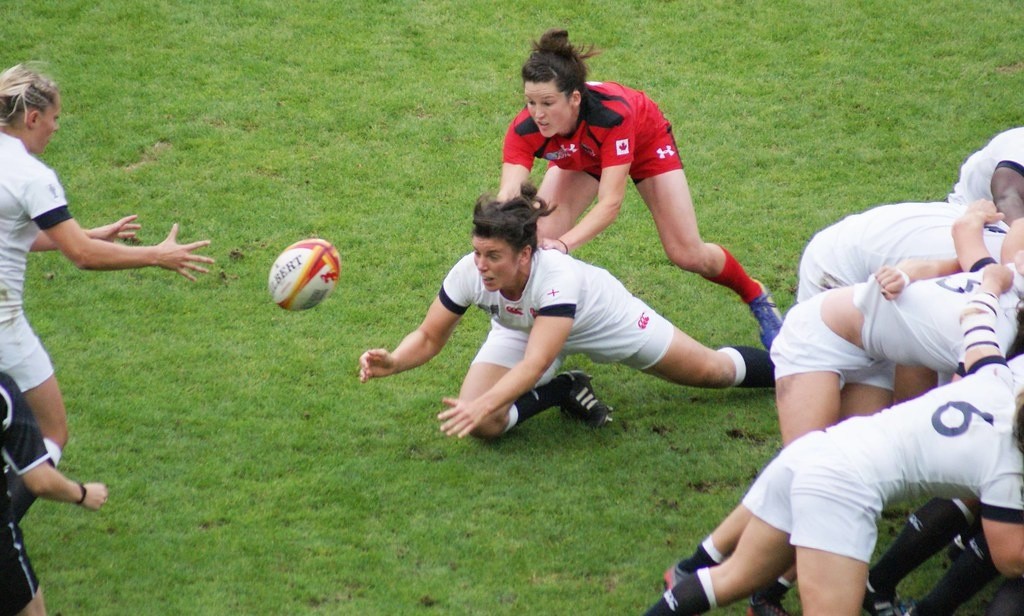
[271,239,340,310]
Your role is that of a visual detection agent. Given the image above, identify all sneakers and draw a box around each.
[861,576,906,616]
[749,599,794,616]
[749,280,782,348]
[563,371,613,428]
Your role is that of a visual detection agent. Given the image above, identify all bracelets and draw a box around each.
[557,238,569,256]
[895,266,910,288]
[77,484,87,506]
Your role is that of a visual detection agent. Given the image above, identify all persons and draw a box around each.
[0,62,215,473]
[0,371,109,616]
[946,126,1023,226]
[643,263,1024,616]
[496,28,783,350]
[357,181,776,441]
[797,202,1024,405]
[861,493,1005,615]
[662,198,1023,616]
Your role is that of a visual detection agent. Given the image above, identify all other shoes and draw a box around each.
[665,560,689,591]
